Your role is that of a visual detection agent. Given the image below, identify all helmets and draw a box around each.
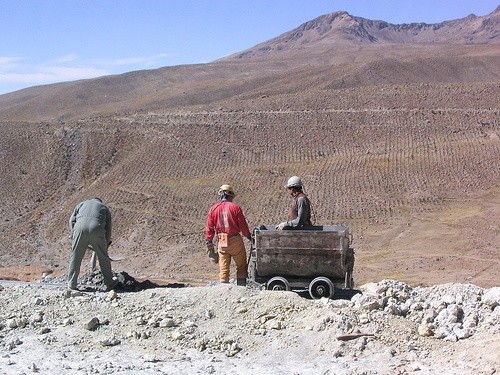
[283,176,303,189]
[217,185,235,199]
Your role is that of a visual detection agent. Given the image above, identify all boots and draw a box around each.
[237,278,246,286]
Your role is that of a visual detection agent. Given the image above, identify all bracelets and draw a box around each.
[286,221,289,226]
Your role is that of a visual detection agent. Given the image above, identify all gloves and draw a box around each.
[206,241,214,257]
[246,236,255,245]
[275,222,289,230]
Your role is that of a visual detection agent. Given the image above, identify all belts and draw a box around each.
[229,233,240,238]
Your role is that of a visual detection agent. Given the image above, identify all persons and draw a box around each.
[205,185,254,286]
[274,177,311,231]
[66,197,119,291]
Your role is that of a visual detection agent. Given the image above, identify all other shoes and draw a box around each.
[107,278,120,290]
